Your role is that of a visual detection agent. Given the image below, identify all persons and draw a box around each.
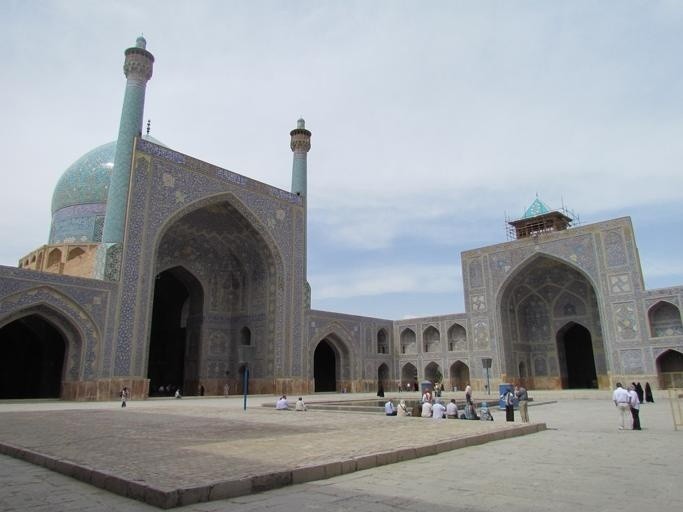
[627,382,642,430]
[280,394,289,409]
[411,399,422,417]
[645,382,655,403]
[431,398,447,418]
[120,386,129,407]
[636,383,644,404]
[384,398,397,416]
[433,382,442,396]
[397,399,411,416]
[200,382,205,396]
[174,388,182,400]
[463,400,480,420]
[499,386,517,422]
[465,381,474,404]
[223,383,229,395]
[227,384,231,395]
[445,398,459,419]
[613,382,633,431]
[275,397,282,408]
[421,387,434,405]
[514,382,530,422]
[295,396,307,411]
[421,399,433,417]
[478,401,495,421]
[397,382,417,393]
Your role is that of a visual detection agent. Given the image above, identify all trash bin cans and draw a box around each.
[499,384,514,409]
[453,387,457,392]
[593,380,598,388]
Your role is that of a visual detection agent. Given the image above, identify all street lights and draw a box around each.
[482,358,492,395]
[238,345,256,410]
